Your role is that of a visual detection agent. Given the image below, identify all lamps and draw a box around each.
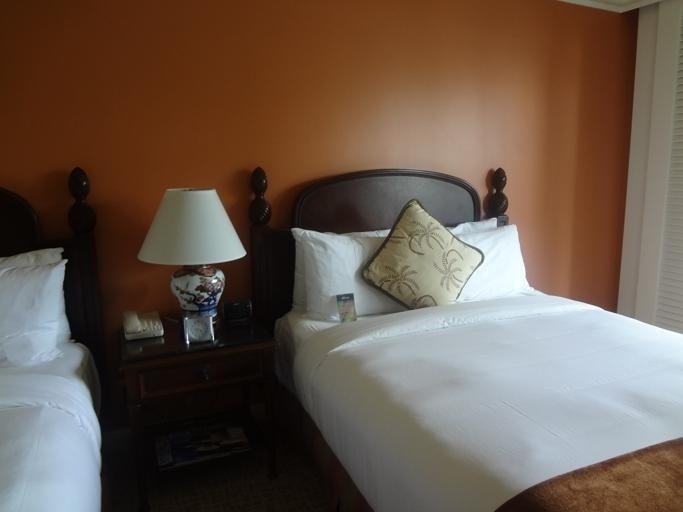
[135,189,248,326]
[119,320,279,510]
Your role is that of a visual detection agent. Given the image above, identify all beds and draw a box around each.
[249,168,683,511]
[0,166,115,508]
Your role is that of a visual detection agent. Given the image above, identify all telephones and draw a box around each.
[121,310,164,340]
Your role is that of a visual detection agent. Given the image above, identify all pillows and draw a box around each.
[290,199,528,321]
[0,242,72,354]
